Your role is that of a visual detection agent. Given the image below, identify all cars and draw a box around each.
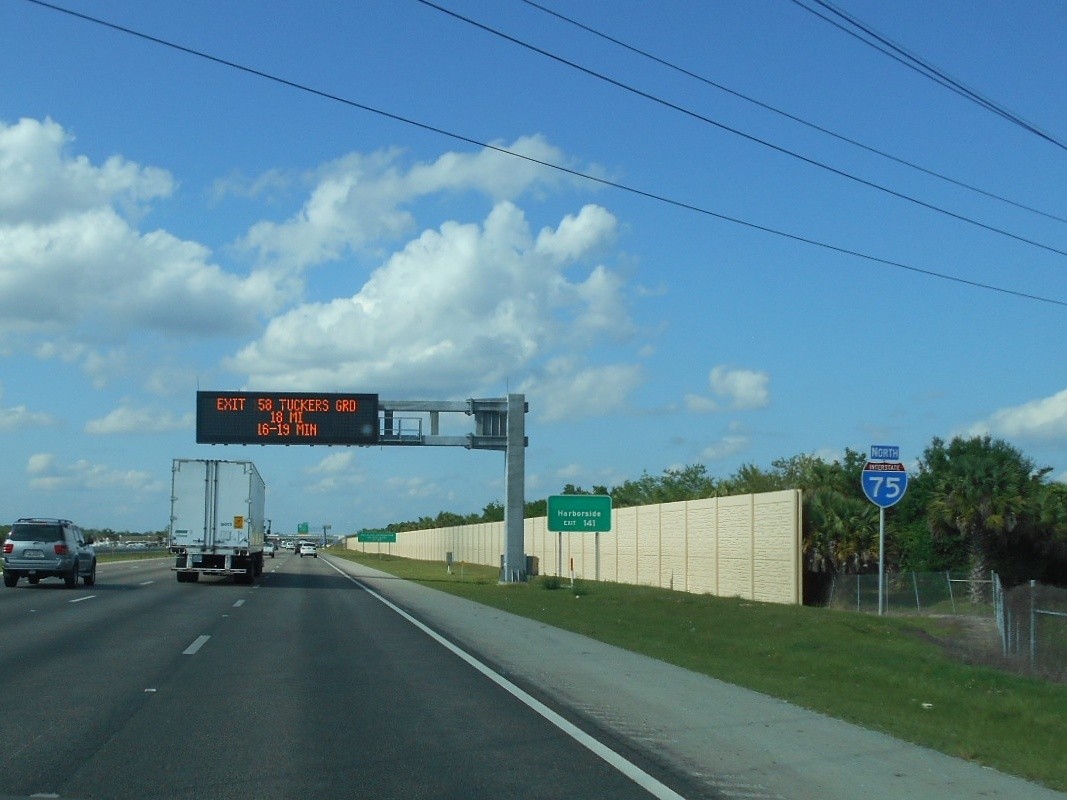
[280,540,303,555]
[299,542,318,558]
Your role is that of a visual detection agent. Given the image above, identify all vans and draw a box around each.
[261,542,275,558]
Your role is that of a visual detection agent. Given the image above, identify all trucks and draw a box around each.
[164,458,272,585]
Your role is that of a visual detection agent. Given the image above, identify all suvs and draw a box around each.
[0,518,98,589]
